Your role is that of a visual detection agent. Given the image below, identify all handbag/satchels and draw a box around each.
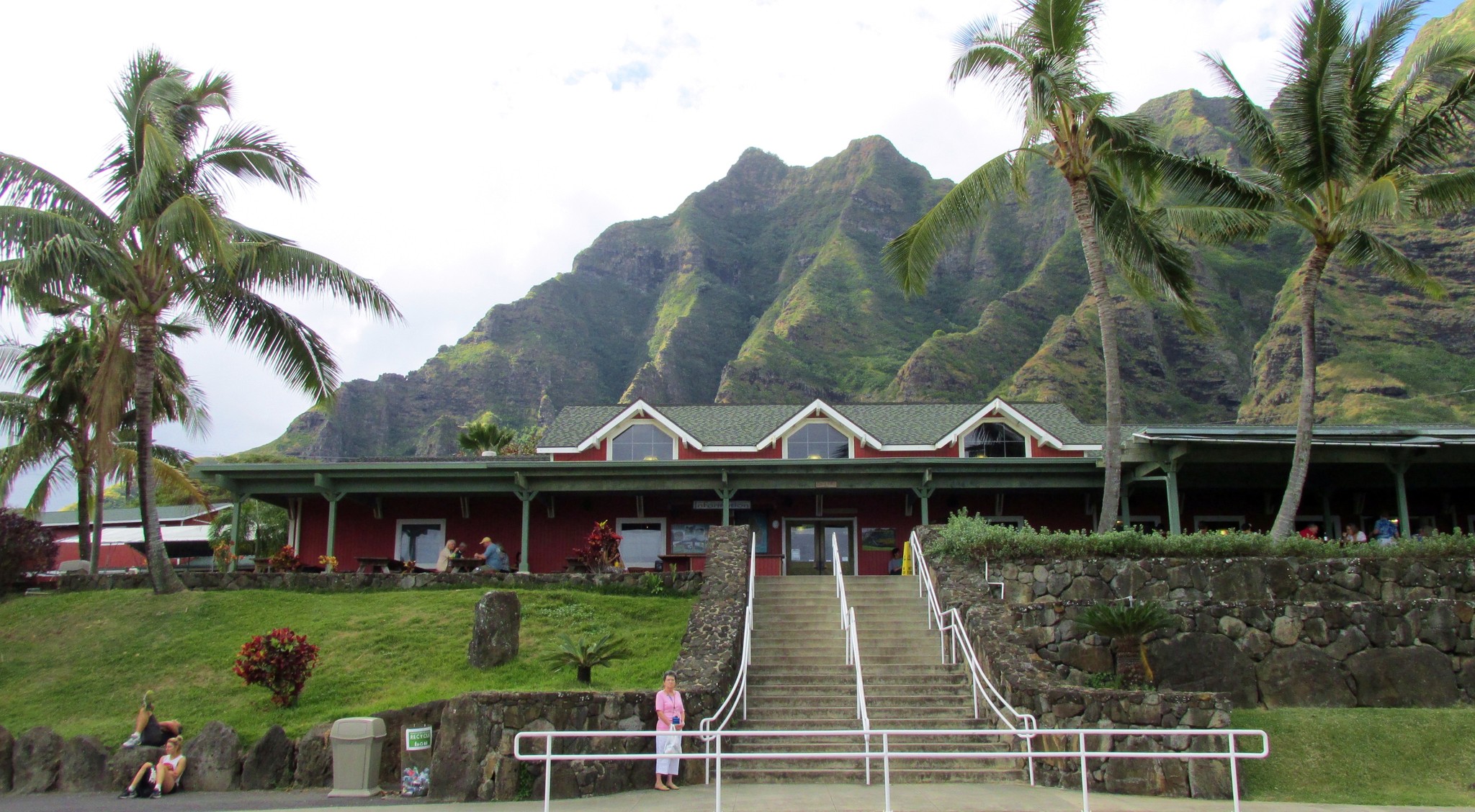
[664,724,681,755]
[452,549,462,558]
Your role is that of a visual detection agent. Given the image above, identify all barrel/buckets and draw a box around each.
[401,723,432,797]
[655,561,663,571]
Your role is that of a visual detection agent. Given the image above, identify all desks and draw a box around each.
[448,557,484,573]
[249,557,275,573]
[356,556,402,574]
[566,556,586,573]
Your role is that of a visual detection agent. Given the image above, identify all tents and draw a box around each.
[52,522,285,574]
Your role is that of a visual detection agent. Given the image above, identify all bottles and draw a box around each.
[403,766,419,781]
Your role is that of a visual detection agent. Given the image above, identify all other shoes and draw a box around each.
[665,783,679,789]
[144,690,155,713]
[122,735,141,748]
[654,782,669,791]
[150,789,162,799]
[118,789,135,799]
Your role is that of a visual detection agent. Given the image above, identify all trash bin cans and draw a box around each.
[328,718,388,797]
[401,723,433,797]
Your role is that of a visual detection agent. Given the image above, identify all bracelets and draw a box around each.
[681,721,685,726]
[516,568,518,570]
[615,555,617,558]
[613,557,615,560]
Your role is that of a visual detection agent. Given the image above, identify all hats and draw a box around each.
[478,537,492,544]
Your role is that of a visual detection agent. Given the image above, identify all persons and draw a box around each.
[601,537,630,573]
[118,736,186,799]
[511,551,532,573]
[472,537,511,574]
[654,670,685,791]
[122,690,183,748]
[1198,520,1209,534]
[1299,523,1319,540]
[450,542,468,573]
[1241,523,1252,533]
[1416,528,1427,542]
[437,539,461,574]
[1342,523,1367,544]
[1367,510,1400,547]
[888,547,902,575]
